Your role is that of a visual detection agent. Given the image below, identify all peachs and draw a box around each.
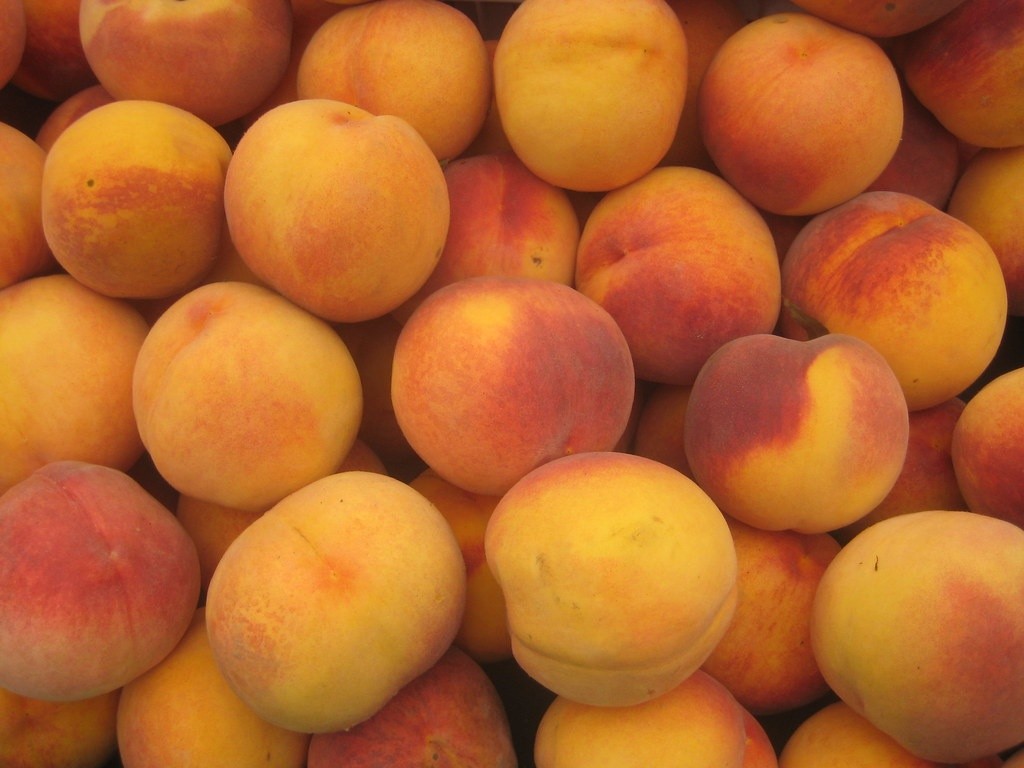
[0,1,1024,768]
